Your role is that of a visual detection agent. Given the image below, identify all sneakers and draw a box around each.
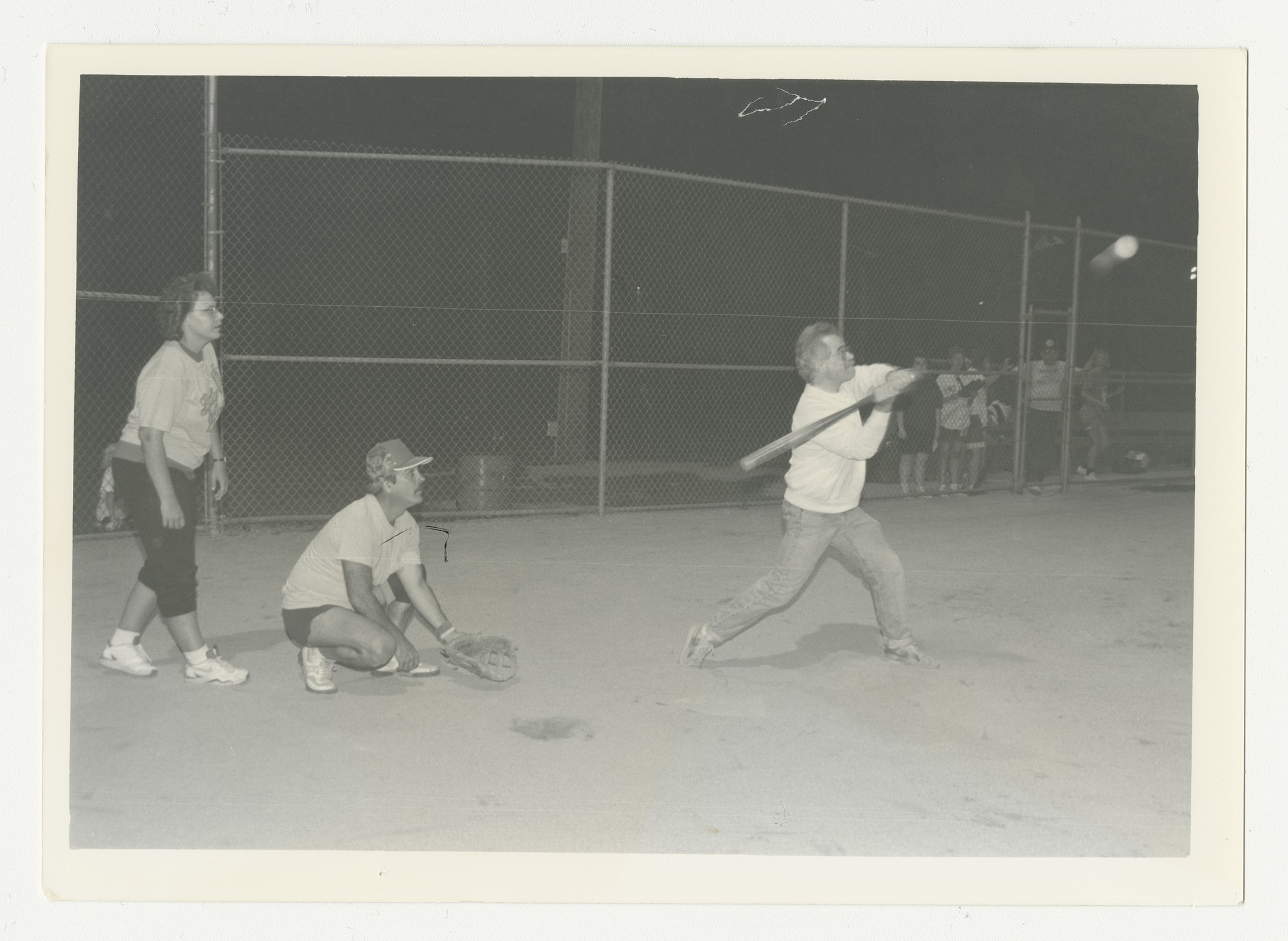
[370,653,439,676]
[99,640,157,678]
[297,645,337,694]
[679,620,724,663]
[184,656,249,685]
[885,630,924,665]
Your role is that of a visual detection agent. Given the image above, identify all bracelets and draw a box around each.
[440,627,456,640]
[212,456,229,463]
[885,368,902,377]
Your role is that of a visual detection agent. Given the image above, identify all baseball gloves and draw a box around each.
[440,631,521,683]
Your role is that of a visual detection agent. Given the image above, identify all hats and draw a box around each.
[365,439,433,479]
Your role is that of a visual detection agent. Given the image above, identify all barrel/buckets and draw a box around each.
[456,453,514,512]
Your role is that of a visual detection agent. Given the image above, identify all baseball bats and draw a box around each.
[739,395,876,473]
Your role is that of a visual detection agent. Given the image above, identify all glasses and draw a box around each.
[187,305,224,318]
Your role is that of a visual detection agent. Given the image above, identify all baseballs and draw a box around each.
[1114,235,1139,259]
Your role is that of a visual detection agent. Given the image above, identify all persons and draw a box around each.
[280,437,520,695]
[95,271,252,689]
[895,342,1113,495]
[677,319,946,670]
